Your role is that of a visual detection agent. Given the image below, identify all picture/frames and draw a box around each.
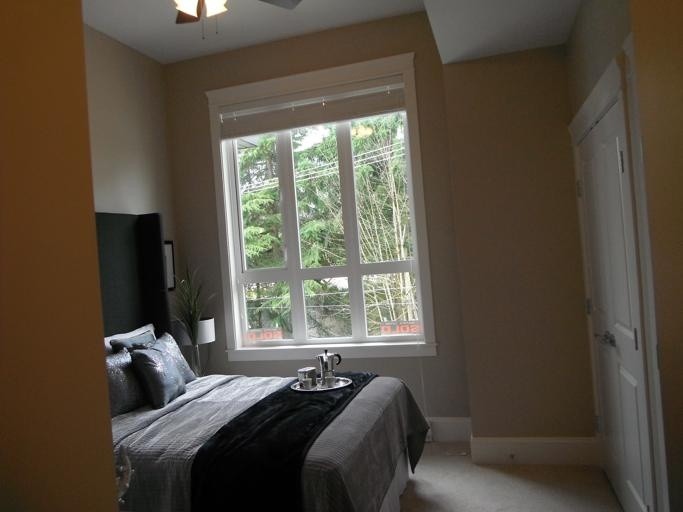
[164,240,176,291]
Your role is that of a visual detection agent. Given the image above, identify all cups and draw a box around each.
[302,378,312,390]
[324,376,336,388]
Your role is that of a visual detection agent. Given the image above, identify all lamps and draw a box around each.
[171,317,216,378]
[174,0,229,42]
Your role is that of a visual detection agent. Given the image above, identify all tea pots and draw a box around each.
[316,349,342,386]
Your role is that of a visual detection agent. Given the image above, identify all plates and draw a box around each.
[289,375,354,392]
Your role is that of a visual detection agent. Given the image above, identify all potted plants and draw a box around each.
[167,255,218,377]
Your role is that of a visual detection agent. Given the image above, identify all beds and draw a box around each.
[110,372,431,512]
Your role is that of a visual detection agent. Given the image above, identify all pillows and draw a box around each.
[103,322,198,421]
